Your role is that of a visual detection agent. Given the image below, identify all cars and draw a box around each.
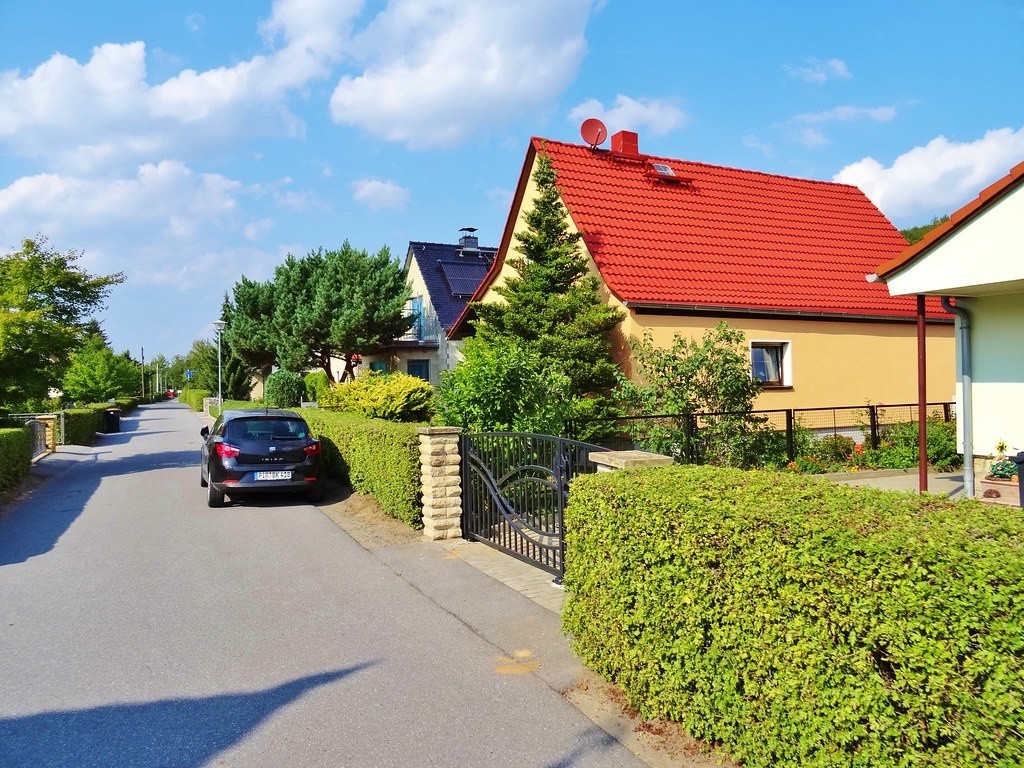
[201,408,321,509]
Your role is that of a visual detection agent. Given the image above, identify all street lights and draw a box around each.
[211,320,228,417]
